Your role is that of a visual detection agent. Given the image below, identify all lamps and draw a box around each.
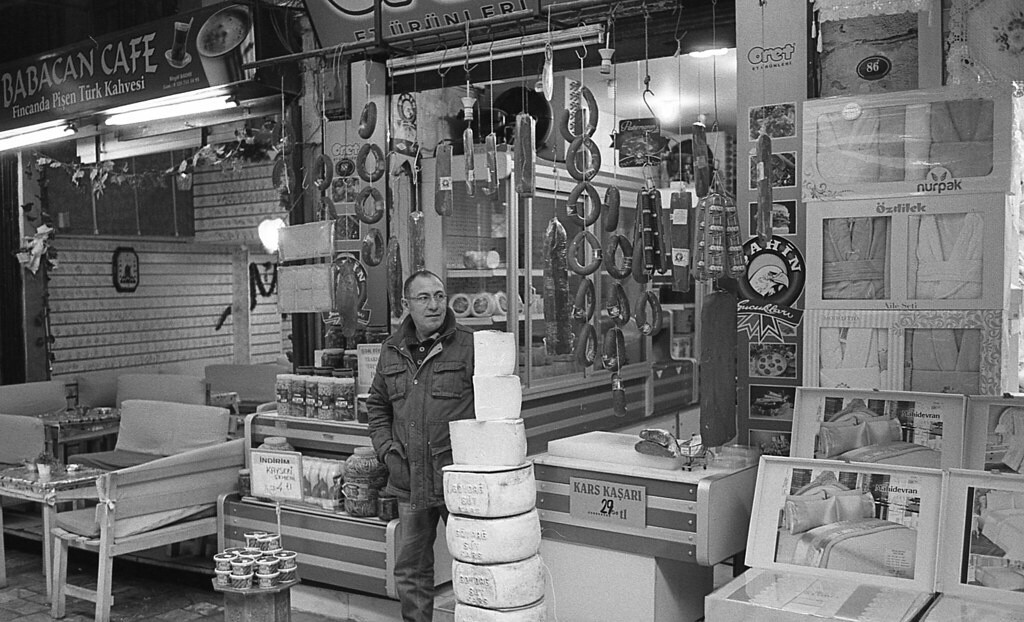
[386,24,605,77]
[0,122,78,153]
[99,95,239,128]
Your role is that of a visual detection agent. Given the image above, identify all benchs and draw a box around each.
[0,363,288,621]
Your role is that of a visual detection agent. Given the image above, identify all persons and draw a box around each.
[365,270,475,622]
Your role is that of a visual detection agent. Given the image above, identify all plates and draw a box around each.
[165,49,191,68]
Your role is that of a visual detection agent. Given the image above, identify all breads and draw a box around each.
[440,329,548,622]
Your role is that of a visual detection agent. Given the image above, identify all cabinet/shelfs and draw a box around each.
[217,402,455,599]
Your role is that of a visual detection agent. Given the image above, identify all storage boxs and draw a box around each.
[703,82,1024,622]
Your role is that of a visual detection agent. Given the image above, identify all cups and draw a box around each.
[197,4,254,89]
[378,498,398,521]
[170,21,191,65]
[213,532,298,590]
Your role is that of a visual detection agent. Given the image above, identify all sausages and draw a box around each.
[270,100,426,320]
[432,80,773,417]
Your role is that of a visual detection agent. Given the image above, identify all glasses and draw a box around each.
[406,292,448,306]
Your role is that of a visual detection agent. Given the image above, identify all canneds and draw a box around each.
[275,349,371,423]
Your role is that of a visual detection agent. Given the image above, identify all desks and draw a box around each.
[0,466,107,602]
[43,407,122,465]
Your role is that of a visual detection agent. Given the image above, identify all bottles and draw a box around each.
[239,469,251,496]
[343,447,389,516]
[275,373,355,422]
